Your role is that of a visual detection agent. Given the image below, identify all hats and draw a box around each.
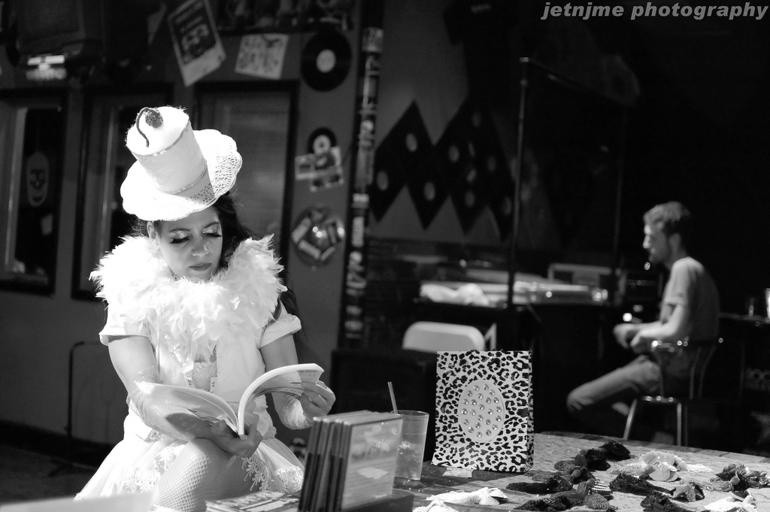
[117,104,243,223]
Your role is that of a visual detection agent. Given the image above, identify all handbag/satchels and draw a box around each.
[432,348,536,475]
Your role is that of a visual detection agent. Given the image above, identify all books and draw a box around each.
[205,408,405,510]
[135,361,325,442]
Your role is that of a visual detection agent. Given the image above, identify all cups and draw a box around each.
[390,410,430,486]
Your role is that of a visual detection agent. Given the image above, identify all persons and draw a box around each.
[567,201,719,439]
[73,106,336,511]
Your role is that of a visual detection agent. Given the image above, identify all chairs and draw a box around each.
[623,334,723,447]
